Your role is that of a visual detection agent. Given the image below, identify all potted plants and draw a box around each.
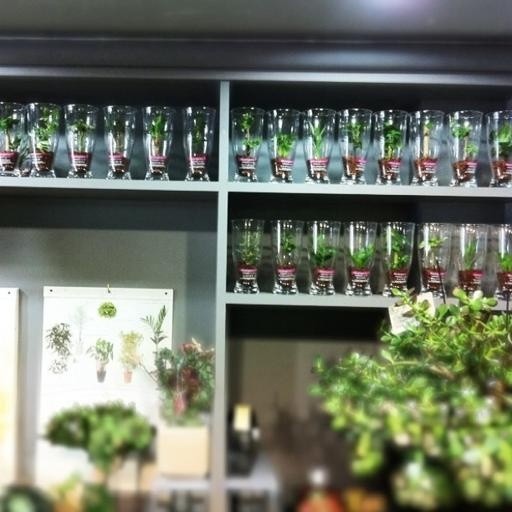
[140,306,216,480]
[86,338,113,383]
[122,330,144,384]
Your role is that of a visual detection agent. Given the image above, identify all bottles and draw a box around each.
[296,468,342,511]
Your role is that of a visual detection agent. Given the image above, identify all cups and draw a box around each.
[230,106,512,189]
[1,99,216,181]
[233,218,511,299]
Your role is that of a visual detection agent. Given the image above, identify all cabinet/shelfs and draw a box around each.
[0,67,511,512]
